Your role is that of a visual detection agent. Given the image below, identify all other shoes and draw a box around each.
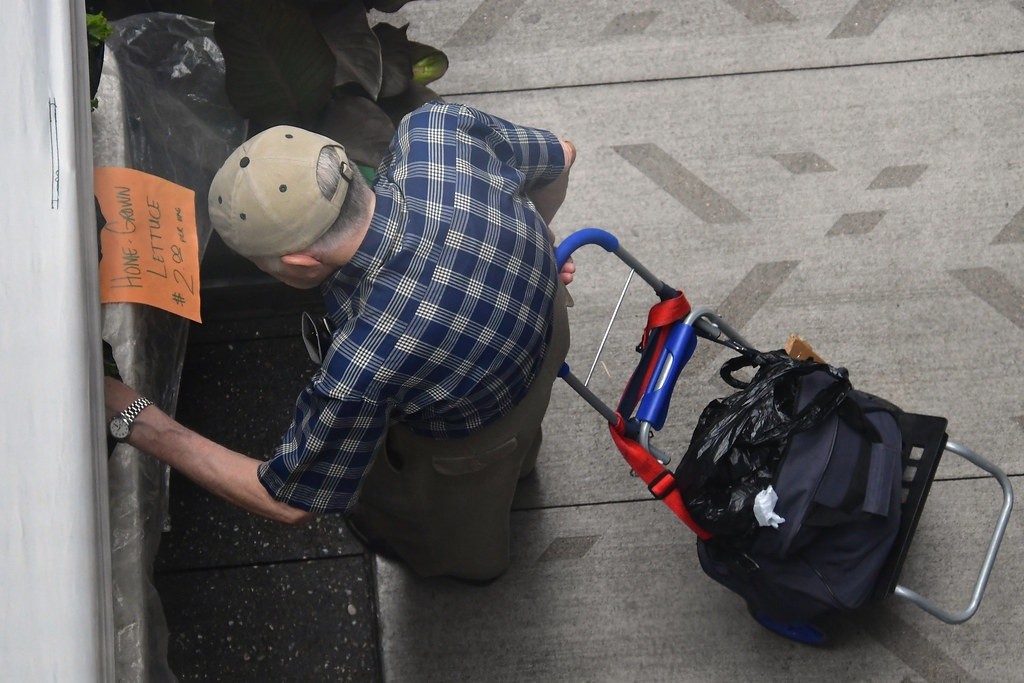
[341,514,382,557]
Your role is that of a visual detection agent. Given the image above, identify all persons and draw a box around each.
[105,101,577,594]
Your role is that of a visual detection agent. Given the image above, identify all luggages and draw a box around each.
[546,228,1014,649]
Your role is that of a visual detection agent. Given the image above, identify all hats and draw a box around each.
[208,126,351,256]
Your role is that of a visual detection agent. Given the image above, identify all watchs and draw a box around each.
[109,396,158,443]
